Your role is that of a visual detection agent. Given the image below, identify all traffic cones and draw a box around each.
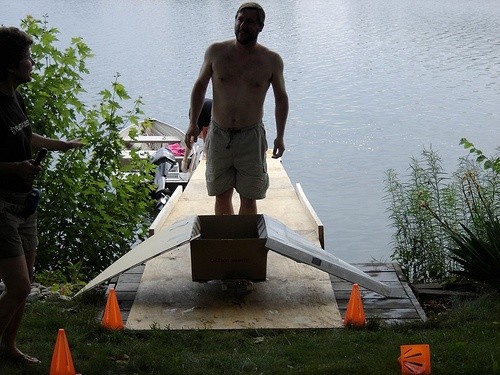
[98,287,126,328]
[49,328,77,375]
[342,283,366,328]
[396,344,431,375]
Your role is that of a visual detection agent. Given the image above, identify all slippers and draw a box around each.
[1,352,42,366]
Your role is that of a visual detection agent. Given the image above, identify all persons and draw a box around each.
[0,26,88,367]
[185,2,289,291]
[187,96,213,161]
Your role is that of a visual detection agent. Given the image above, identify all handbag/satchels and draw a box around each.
[23,188,39,216]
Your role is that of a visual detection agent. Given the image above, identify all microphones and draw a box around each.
[33,148,48,166]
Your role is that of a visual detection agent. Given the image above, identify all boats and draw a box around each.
[113,116,196,211]
